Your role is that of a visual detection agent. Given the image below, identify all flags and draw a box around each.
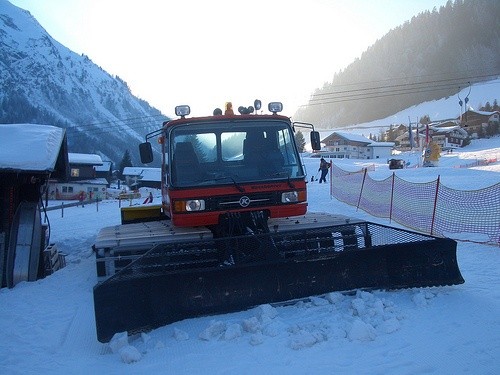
[408,120,414,149]
[426,124,429,143]
[416,120,420,147]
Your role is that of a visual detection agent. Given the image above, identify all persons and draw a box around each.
[318,158,331,183]
[149,191,154,203]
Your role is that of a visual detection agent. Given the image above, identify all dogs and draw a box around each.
[310,176,316,181]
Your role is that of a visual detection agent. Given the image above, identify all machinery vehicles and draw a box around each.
[91,100,467,342]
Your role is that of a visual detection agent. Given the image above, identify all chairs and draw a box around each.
[242,137,285,182]
[170,142,208,187]
